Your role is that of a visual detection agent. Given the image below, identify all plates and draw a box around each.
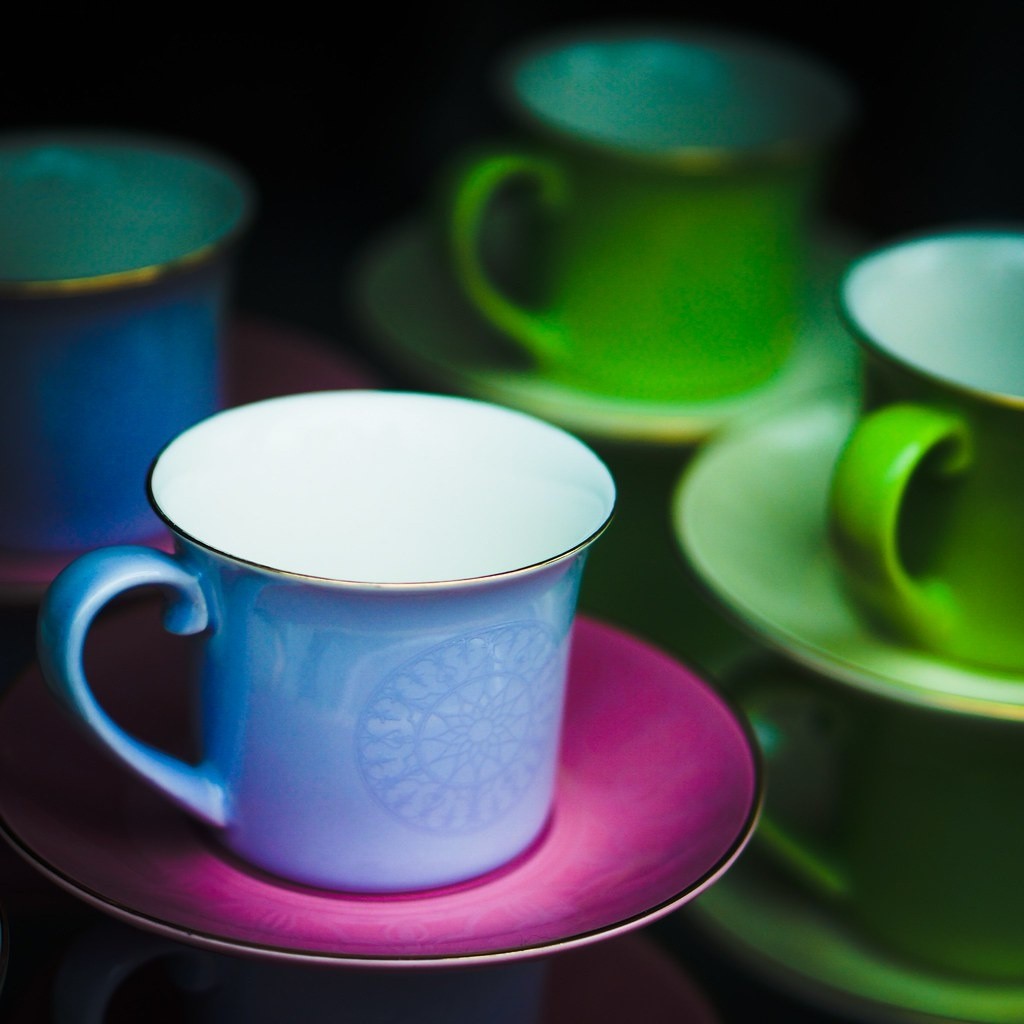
[0,600,767,968]
[0,302,373,606]
[669,371,1024,726]
[359,188,894,450]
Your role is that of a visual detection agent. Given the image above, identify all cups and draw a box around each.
[41,389,621,895]
[826,228,1024,674]
[444,36,843,408]
[0,130,248,554]
